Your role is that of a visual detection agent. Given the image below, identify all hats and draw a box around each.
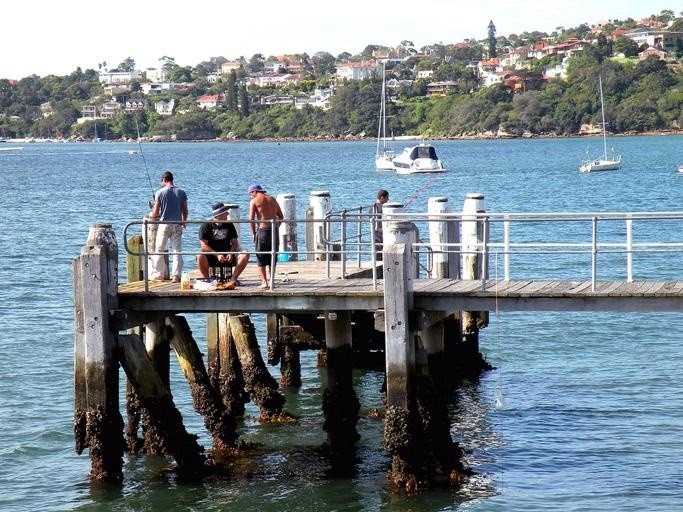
[212,203,229,218]
[248,184,267,193]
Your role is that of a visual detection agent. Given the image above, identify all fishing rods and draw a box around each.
[134,118,155,209]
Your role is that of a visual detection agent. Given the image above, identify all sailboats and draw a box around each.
[578,72,622,173]
[375,62,396,171]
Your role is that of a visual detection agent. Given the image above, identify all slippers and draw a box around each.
[235,281,245,286]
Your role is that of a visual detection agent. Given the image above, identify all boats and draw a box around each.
[391,143,449,176]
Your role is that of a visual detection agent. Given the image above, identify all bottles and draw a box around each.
[181,273,191,290]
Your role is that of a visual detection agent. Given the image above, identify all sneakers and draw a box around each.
[171,275,180,283]
[153,273,165,279]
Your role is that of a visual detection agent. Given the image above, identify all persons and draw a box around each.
[196,202,250,286]
[149,172,189,283]
[248,184,284,288]
[369,190,389,260]
